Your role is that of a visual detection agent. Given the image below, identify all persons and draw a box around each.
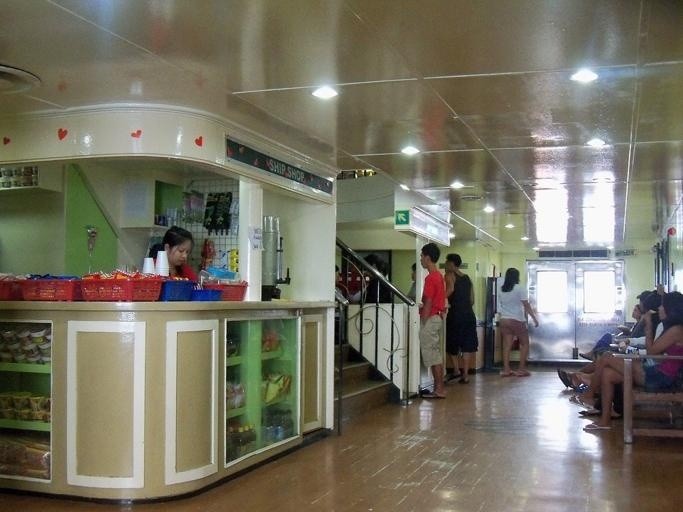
[147,224,200,285]
[360,254,394,383]
[402,262,416,303]
[553,288,683,432]
[337,256,369,303]
[443,252,478,384]
[335,264,358,344]
[495,266,540,378]
[416,241,450,400]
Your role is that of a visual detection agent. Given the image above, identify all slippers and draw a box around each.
[583,421,613,431]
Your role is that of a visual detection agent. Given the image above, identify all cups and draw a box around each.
[142,251,169,277]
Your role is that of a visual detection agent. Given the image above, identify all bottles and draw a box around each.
[227,424,257,457]
[265,410,293,443]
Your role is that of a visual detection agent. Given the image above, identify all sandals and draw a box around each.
[558,369,601,416]
[422,391,446,399]
[444,372,469,384]
[502,369,530,377]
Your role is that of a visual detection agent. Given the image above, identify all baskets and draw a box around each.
[0,279,247,302]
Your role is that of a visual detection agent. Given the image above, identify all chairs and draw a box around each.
[610,343,683,443]
